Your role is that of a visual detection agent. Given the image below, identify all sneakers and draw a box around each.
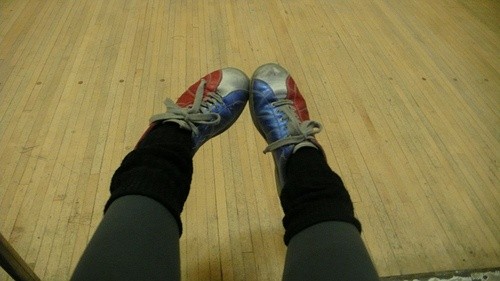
[149,67,250,159]
[249,63,328,199]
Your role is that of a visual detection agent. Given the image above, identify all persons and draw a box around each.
[72,63,381,281]
